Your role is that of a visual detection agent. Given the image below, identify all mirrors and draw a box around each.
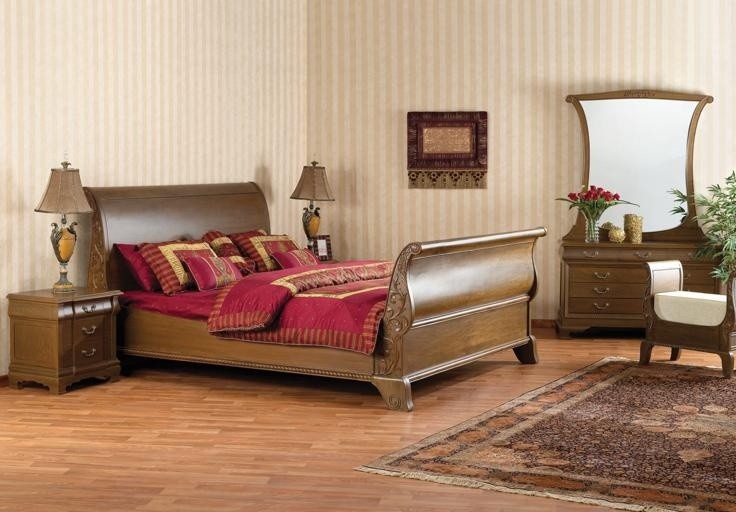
[566,91,709,239]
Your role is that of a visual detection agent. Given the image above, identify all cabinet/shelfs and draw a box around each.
[554,241,733,337]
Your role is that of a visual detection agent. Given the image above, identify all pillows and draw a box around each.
[273,251,325,270]
[140,241,226,298]
[231,229,270,273]
[187,257,245,291]
[112,241,152,294]
[202,228,248,279]
[237,235,303,271]
[135,264,159,267]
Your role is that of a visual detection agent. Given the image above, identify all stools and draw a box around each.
[641,257,735,376]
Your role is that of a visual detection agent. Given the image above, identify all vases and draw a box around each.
[573,206,609,244]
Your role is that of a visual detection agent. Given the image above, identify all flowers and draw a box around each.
[556,182,635,208]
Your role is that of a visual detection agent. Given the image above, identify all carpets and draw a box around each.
[354,355,733,507]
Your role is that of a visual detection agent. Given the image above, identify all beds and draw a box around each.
[82,180,550,413]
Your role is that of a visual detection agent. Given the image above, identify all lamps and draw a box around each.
[33,161,87,293]
[289,158,339,261]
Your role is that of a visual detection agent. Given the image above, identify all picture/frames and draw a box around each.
[311,233,335,265]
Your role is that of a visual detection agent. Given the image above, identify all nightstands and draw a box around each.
[5,290,124,392]
